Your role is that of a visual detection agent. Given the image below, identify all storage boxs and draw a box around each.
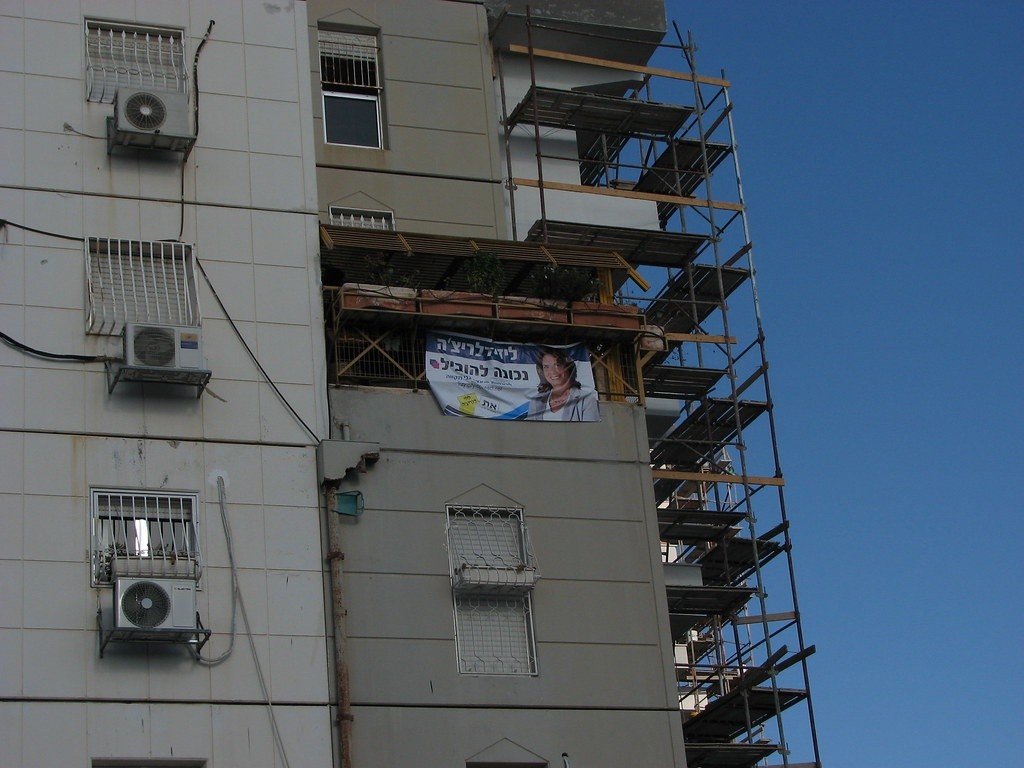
[458,566,534,595]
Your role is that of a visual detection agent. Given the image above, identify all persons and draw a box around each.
[526,350,600,421]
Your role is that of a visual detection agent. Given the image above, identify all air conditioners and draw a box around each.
[110,87,194,153]
[122,320,203,383]
[112,577,197,643]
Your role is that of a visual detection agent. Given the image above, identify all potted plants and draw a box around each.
[106,541,197,581]
[336,249,640,342]
